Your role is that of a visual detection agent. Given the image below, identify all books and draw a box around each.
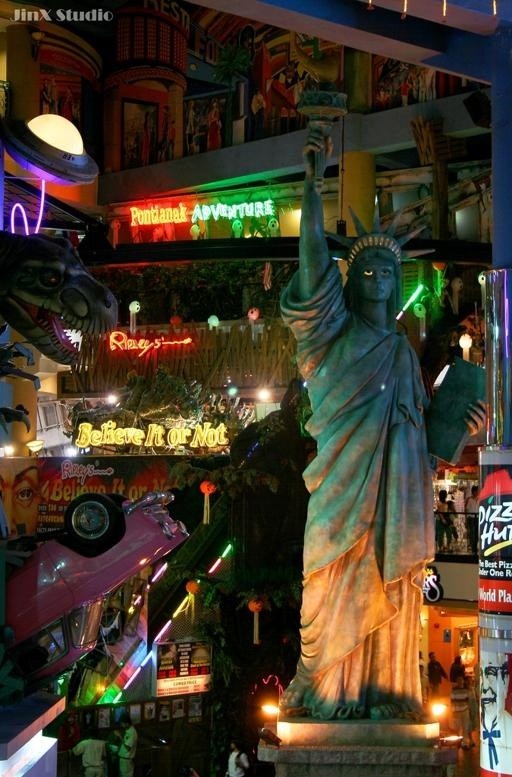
[423,358,491,468]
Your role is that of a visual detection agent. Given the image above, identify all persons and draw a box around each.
[272,124,494,723]
[447,498,461,549]
[463,485,480,556]
[417,649,480,752]
[70,727,110,777]
[57,711,80,752]
[112,712,138,777]
[223,739,250,777]
[434,489,454,552]
